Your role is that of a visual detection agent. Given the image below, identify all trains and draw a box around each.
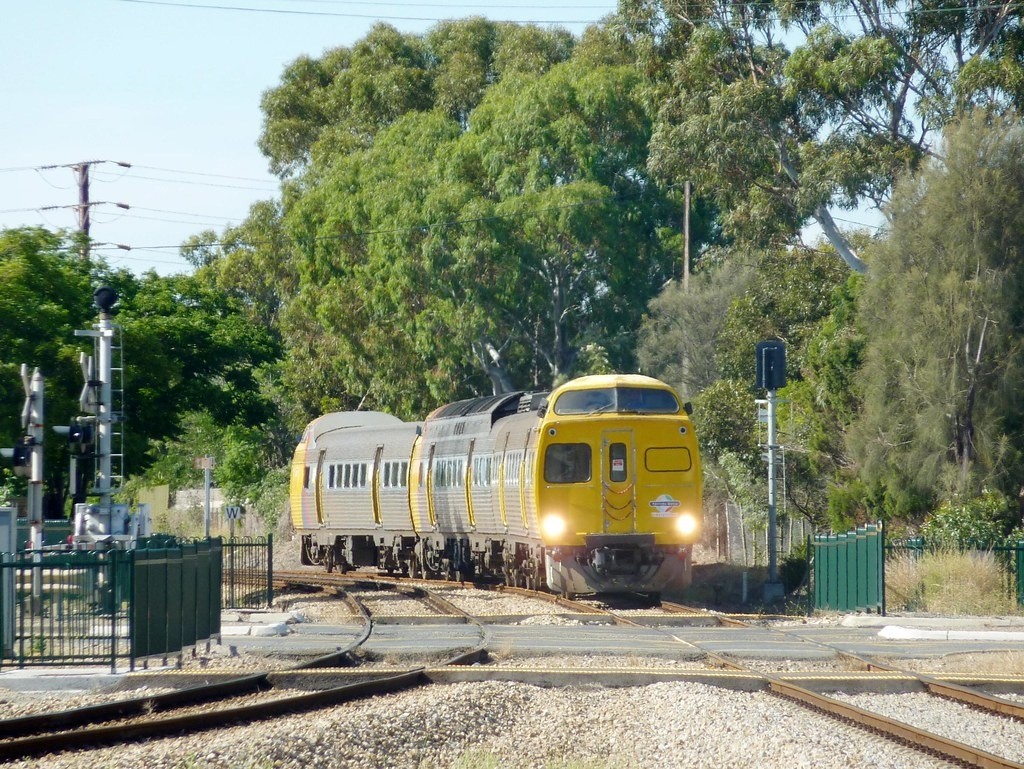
[290,374,704,600]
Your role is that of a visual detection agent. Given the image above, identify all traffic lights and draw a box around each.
[53,424,94,444]
[13,445,31,467]
